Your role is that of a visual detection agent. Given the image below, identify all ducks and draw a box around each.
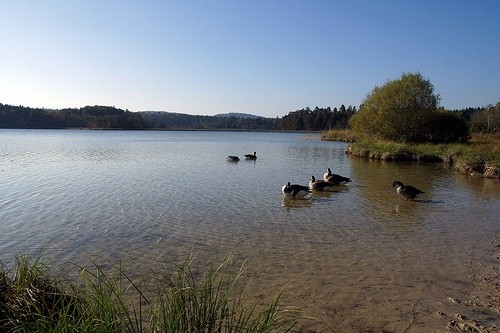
[226,155,241,162]
[391,181,425,201]
[244,151,257,161]
[324,167,352,185]
[308,175,334,192]
[280,182,311,199]
